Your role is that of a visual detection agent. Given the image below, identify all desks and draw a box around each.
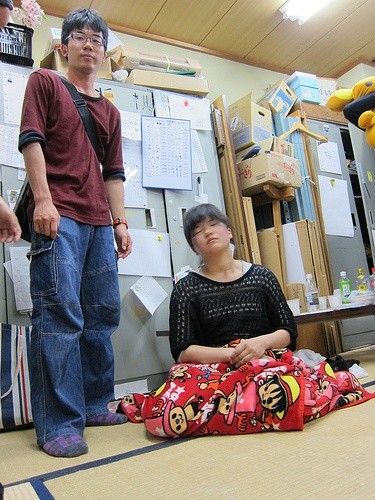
[155,305,374,364]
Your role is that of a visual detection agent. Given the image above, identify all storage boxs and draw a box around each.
[40,27,208,98]
[228,70,320,192]
[0,22,35,66]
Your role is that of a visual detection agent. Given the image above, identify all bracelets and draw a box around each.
[113,217,128,229]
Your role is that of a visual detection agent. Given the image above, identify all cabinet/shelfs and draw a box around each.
[302,115,375,294]
[0,60,225,402]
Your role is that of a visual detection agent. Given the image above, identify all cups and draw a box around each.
[328,295,339,307]
[286,299,300,316]
[318,295,327,310]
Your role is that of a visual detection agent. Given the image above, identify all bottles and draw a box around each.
[304,273,320,313]
[356,267,369,296]
[369,267,375,294]
[339,271,352,304]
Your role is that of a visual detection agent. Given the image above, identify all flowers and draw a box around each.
[11,0,47,29]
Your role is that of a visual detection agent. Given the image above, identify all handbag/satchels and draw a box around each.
[13,172,36,244]
[0,322,34,432]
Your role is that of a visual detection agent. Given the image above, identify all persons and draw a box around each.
[0,0,23,243]
[152,204,305,438]
[19,7,133,457]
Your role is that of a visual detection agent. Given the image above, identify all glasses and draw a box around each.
[64,32,107,49]
